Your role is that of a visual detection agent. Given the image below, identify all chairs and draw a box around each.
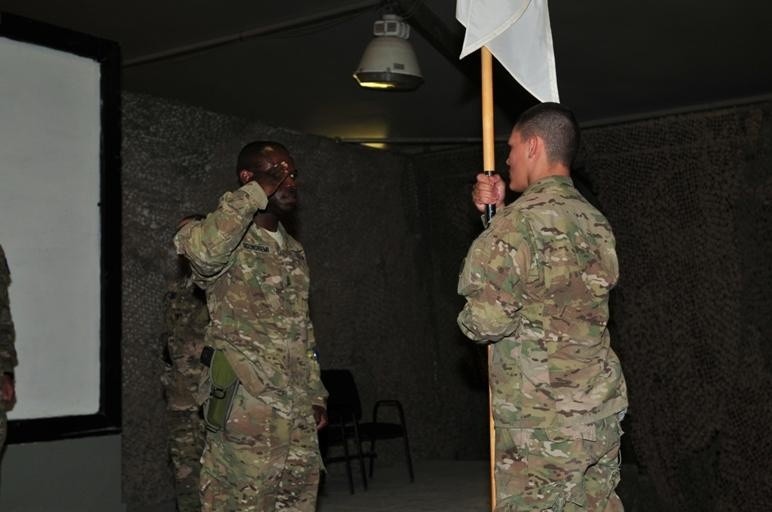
[321,368,364,499]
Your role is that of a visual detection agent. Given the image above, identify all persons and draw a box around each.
[453,101,629,510]
[159,215,211,512]
[1,243,20,459]
[174,139,333,511]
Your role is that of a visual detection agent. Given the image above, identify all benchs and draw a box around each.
[316,399,415,494]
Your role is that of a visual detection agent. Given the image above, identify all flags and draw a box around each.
[453,0,562,109]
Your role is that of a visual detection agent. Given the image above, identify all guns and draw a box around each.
[199,345,239,433]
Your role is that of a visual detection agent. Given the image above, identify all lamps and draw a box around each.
[352,11,425,94]
